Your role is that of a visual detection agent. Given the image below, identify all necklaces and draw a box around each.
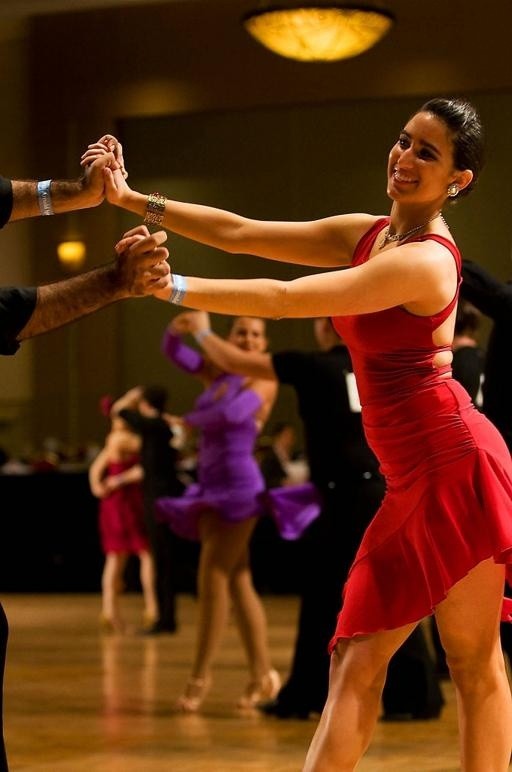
[378,212,450,250]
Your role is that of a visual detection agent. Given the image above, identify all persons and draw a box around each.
[0,132,171,772]
[81,99,512,772]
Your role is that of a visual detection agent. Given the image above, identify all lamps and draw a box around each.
[242,0,396,63]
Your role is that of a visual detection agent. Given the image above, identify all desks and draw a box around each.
[2,474,145,591]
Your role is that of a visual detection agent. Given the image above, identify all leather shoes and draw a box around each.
[376,707,444,723]
[236,669,283,710]
[96,613,180,637]
[257,701,322,719]
[179,676,213,715]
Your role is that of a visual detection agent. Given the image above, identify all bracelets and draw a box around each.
[143,192,167,226]
[37,180,54,216]
